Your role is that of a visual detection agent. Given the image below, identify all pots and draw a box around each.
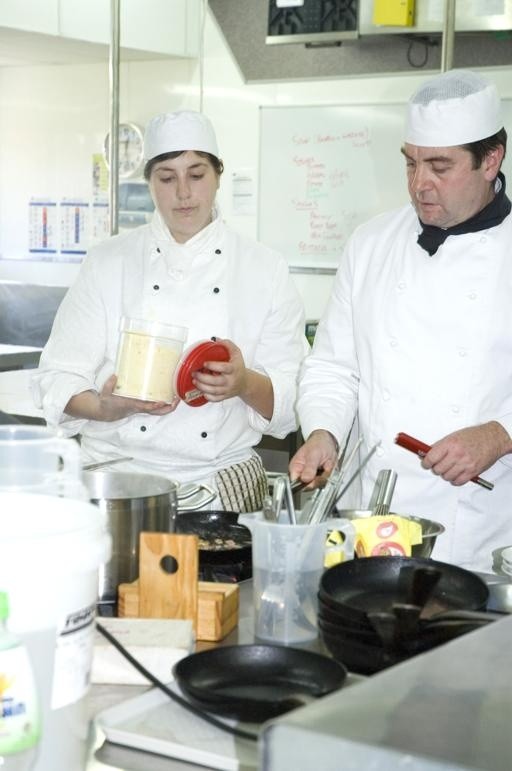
[171,643,347,725]
[82,468,217,605]
[314,556,511,675]
[176,506,252,568]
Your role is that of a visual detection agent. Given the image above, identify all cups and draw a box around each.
[0,423,81,497]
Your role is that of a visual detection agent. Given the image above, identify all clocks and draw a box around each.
[104,120,146,180]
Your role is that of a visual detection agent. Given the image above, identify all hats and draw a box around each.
[140,111,220,178]
[404,68,505,147]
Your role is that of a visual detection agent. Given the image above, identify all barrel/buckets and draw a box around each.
[0,492,115,710]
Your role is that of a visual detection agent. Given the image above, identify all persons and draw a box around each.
[32,113,316,513]
[285,78,512,574]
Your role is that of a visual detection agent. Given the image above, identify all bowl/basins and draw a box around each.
[325,508,444,559]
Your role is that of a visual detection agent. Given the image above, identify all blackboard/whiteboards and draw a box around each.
[258,103,412,274]
